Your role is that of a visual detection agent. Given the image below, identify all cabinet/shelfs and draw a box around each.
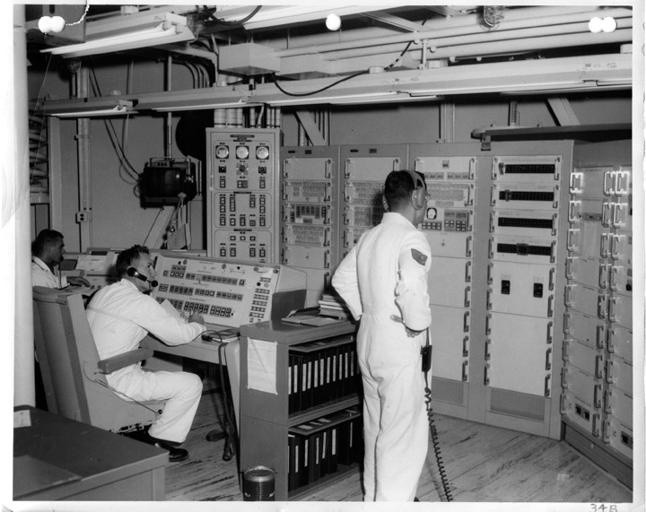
[238,310,364,502]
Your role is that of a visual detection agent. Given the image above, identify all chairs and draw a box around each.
[31,283,167,433]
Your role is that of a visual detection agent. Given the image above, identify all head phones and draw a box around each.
[381,169,432,210]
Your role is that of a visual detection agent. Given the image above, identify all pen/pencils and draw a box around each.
[190,308,193,316]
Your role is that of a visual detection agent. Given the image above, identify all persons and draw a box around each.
[331,171,430,501]
[30,228,90,409]
[89,243,206,461]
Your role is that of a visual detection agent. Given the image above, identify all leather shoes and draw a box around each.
[150,441,188,462]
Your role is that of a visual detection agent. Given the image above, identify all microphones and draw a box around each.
[127,267,158,287]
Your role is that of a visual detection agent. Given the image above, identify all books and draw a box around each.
[286,294,363,492]
